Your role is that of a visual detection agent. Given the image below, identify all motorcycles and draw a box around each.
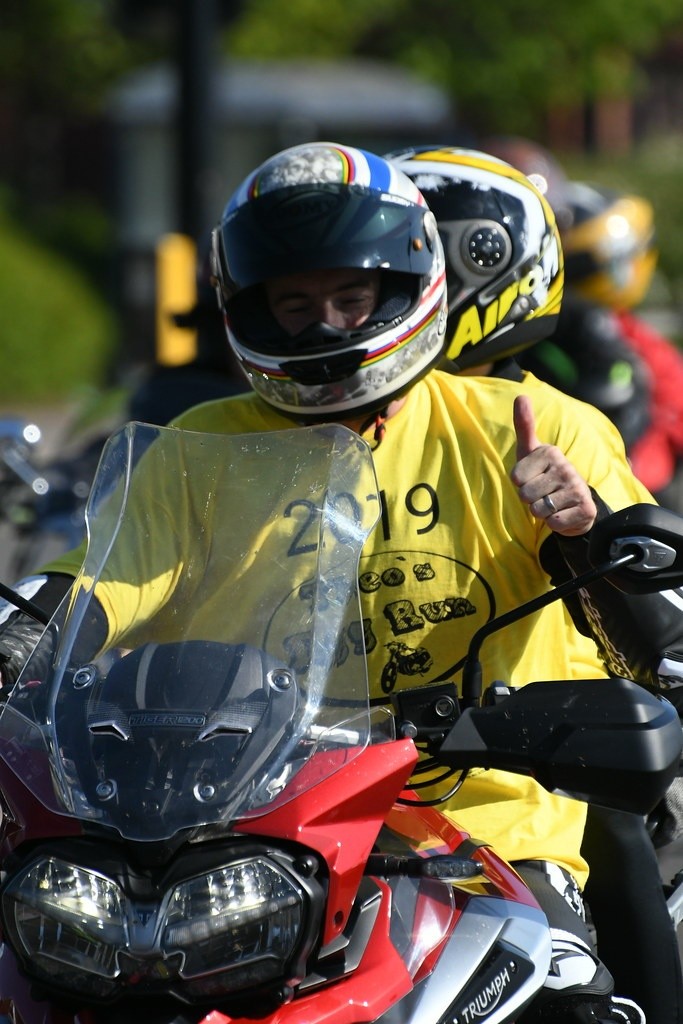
[0,414,683,1024]
[0,390,140,596]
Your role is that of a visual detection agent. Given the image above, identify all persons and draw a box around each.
[381,146,657,506]
[491,152,679,502]
[1,142,680,1024]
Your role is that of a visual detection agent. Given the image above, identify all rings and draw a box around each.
[541,495,558,514]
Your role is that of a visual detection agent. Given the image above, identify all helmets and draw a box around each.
[549,175,659,312]
[208,140,450,423]
[375,145,565,376]
